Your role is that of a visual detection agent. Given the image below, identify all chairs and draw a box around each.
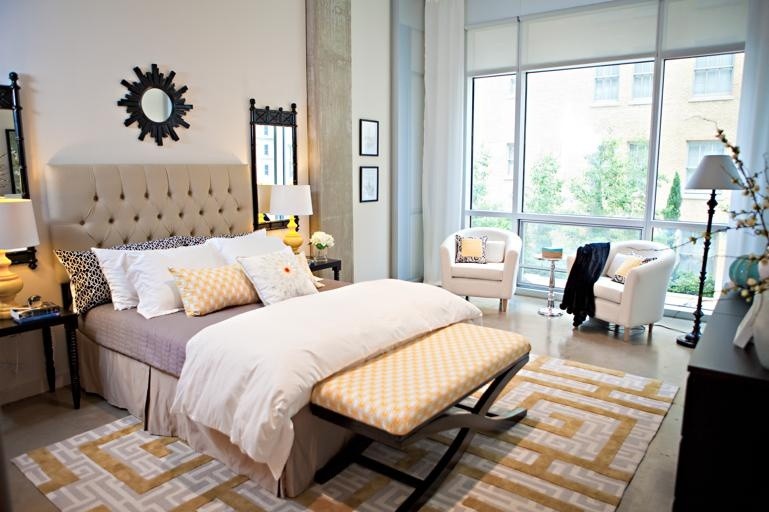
[439,226,524,316]
[564,238,682,347]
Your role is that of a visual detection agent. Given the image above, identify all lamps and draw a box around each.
[0,194,42,314]
[256,186,273,222]
[677,155,746,353]
[268,182,316,255]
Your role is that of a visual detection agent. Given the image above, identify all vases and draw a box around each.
[316,247,327,262]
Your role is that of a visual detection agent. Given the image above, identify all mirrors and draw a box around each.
[250,94,298,233]
[0,70,36,272]
[116,61,195,146]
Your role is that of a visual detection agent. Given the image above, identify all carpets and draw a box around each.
[13,346,682,512]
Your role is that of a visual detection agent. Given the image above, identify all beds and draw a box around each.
[35,156,485,500]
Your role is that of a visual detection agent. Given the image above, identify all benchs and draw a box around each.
[307,322,531,510]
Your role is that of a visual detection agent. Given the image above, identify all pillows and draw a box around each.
[612,254,657,285]
[54,230,324,321]
[455,233,488,265]
[606,253,627,277]
[486,240,505,263]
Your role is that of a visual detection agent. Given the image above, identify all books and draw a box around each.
[9,301,61,324]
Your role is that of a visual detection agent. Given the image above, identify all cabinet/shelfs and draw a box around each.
[674,279,769,511]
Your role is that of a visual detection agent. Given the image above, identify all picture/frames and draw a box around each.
[359,117,380,156]
[359,165,379,203]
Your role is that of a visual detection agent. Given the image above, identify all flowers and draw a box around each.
[648,123,768,300]
[310,232,335,249]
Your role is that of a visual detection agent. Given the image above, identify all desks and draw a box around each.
[308,256,342,281]
[531,252,566,317]
[0,301,82,424]
